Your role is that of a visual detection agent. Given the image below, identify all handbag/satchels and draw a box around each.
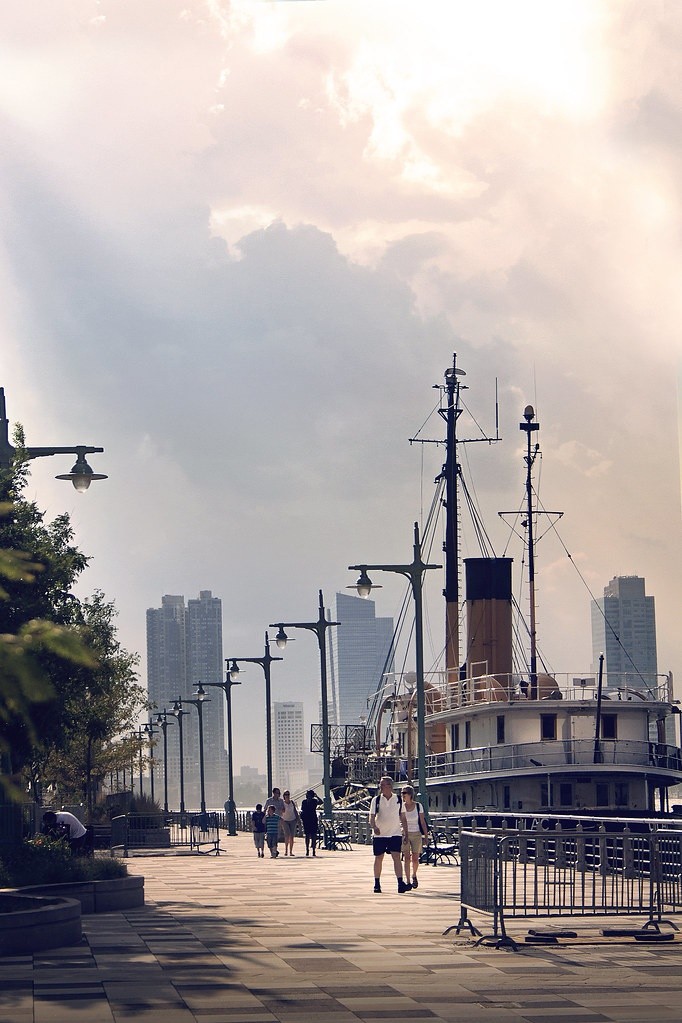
[415,802,430,834]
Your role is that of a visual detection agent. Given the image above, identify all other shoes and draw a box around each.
[374,883,381,892]
[412,877,418,888]
[397,881,407,893]
[407,883,412,890]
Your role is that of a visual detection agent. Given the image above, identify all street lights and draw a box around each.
[110,710,190,829]
[192,680,238,835]
[268,621,338,849]
[225,657,274,798]
[169,698,210,832]
[348,562,444,827]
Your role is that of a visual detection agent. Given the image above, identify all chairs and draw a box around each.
[419,828,460,866]
[306,818,354,854]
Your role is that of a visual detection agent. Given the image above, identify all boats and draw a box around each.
[332,347,682,832]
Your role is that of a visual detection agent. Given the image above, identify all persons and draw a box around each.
[224,796,237,830]
[398,785,428,889]
[262,805,287,858]
[41,811,87,858]
[368,776,412,894]
[279,790,299,856]
[263,788,285,857]
[300,790,324,856]
[251,804,267,858]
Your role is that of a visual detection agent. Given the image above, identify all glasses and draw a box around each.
[400,792,408,795]
[284,793,290,796]
